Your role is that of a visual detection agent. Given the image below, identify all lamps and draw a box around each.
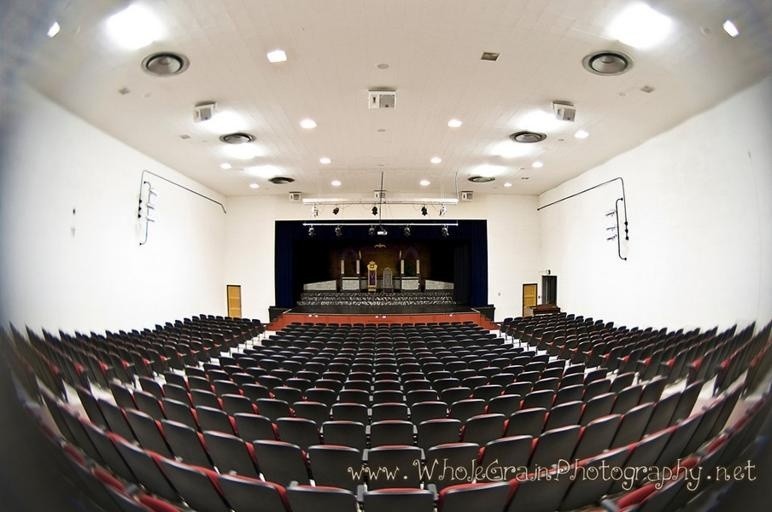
[300,197,461,240]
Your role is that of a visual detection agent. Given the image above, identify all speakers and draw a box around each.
[195,102,216,121]
[553,102,576,122]
[374,190,386,203]
[368,91,397,108]
[289,192,301,201]
[461,191,473,201]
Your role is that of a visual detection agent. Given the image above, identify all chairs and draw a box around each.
[1,285,771,512]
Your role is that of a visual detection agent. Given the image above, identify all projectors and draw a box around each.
[377,231,387,236]
